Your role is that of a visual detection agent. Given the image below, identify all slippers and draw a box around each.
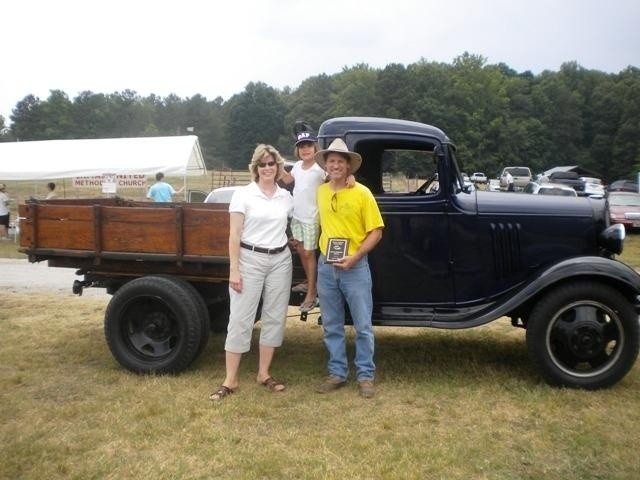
[291,278,309,293]
[257,376,286,393]
[210,383,238,401]
[299,297,319,313]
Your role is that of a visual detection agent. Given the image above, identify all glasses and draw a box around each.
[256,161,276,167]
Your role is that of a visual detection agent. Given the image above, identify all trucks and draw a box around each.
[16,112,640,393]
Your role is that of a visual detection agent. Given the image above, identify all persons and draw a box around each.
[209,143,294,399]
[45,183,57,199]
[505,171,514,191]
[539,173,549,183]
[288,138,386,396]
[281,132,355,312]
[146,172,184,202]
[0,184,11,241]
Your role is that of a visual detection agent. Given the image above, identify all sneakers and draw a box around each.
[357,380,376,397]
[314,375,347,392]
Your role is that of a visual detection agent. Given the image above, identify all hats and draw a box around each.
[312,137,363,176]
[295,130,317,144]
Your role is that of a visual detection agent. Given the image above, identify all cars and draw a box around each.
[426,163,640,234]
[188,185,245,207]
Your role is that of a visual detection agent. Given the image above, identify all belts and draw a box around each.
[241,242,288,255]
